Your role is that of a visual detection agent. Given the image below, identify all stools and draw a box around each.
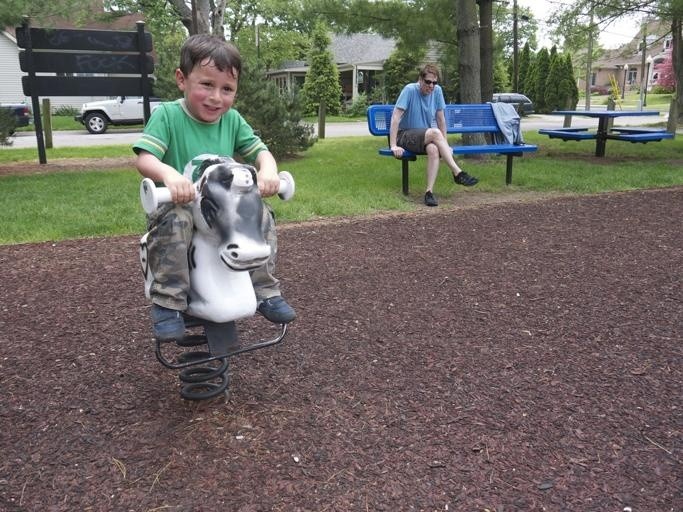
[538,126,675,144]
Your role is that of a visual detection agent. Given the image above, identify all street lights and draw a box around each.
[621,64,628,99]
[644,55,653,106]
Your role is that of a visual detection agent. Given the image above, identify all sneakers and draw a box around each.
[257,297,296,323]
[423,190,438,206]
[452,171,479,186]
[153,306,184,341]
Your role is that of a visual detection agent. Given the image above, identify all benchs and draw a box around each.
[366,104,537,194]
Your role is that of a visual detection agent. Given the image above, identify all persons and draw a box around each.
[389,63,480,207]
[132,34,298,342]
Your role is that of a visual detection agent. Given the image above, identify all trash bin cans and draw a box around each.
[608,99,615,110]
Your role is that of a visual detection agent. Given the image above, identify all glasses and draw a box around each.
[423,79,438,85]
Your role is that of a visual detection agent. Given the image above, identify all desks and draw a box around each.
[552,111,660,157]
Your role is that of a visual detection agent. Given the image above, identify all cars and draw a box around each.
[0,102,31,135]
[493,93,535,113]
[75,95,163,134]
